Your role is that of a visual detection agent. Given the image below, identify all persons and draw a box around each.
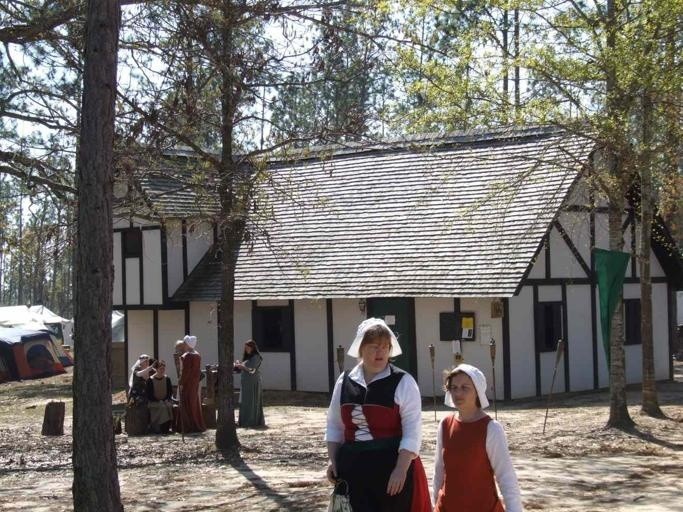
[430,363,525,512]
[234,339,266,428]
[173,335,205,431]
[125,354,175,434]
[322,317,435,512]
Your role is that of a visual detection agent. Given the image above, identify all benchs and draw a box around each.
[124,400,212,437]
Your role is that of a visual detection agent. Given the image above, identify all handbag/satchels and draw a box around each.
[327,480,352,512]
